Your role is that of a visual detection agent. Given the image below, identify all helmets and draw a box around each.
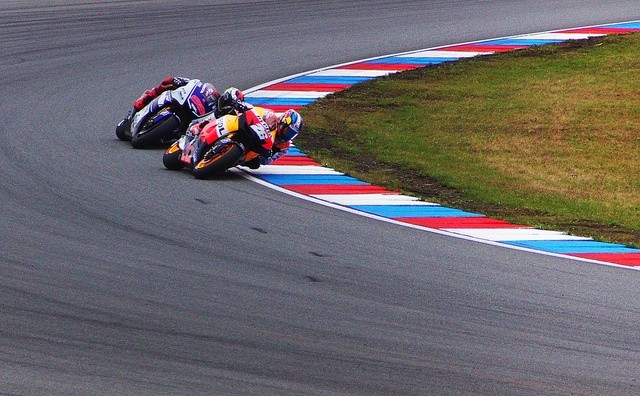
[277,108,304,143]
[218,88,244,112]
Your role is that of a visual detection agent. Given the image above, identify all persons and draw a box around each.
[133,75,246,112]
[190,108,303,167]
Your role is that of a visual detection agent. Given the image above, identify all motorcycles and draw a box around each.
[163,103,271,180]
[116,79,220,148]
